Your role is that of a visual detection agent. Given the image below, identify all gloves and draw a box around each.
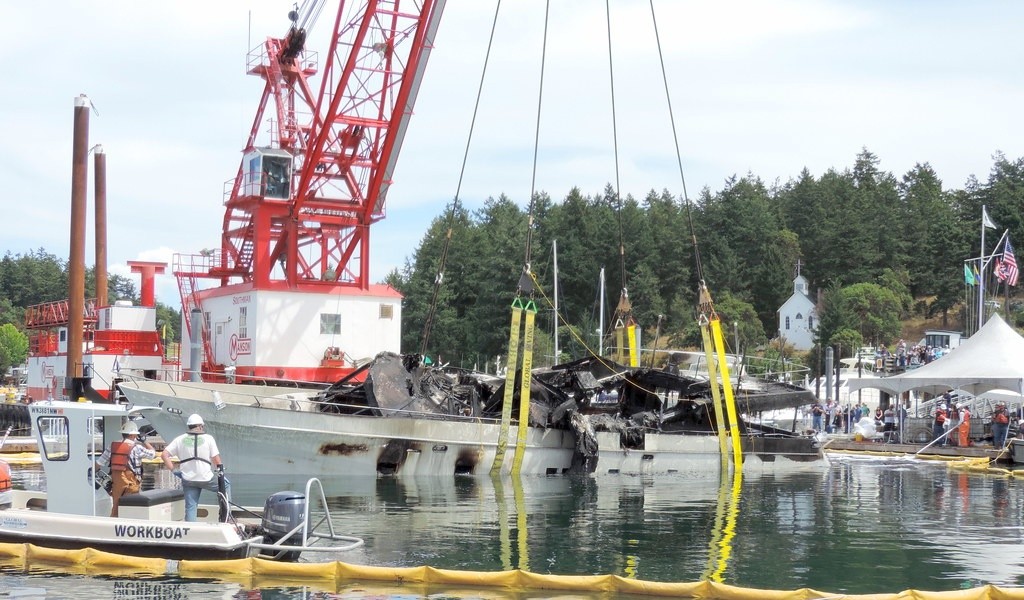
[171,468,182,478]
[137,431,146,443]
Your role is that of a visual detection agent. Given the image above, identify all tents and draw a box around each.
[844,311,1024,442]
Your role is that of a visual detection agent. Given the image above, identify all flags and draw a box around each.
[983,211,997,229]
[965,256,1006,286]
[1004,238,1020,287]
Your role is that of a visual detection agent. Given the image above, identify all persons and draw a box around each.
[161,413,238,525]
[94,421,156,517]
[811,342,1024,450]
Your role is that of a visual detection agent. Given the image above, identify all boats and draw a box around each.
[113,351,838,475]
[0,351,371,562]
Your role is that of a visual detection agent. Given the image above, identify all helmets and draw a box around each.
[940,404,947,409]
[118,421,141,434]
[956,403,962,409]
[999,402,1005,406]
[187,414,205,426]
